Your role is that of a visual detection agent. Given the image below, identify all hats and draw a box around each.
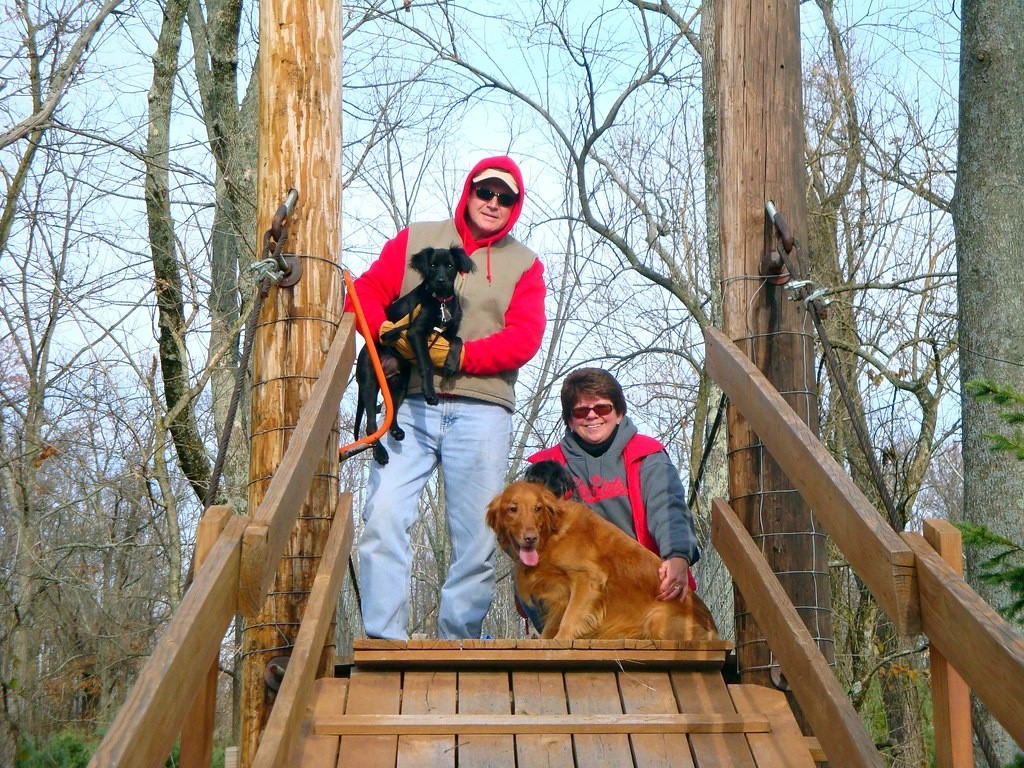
[472,167,520,196]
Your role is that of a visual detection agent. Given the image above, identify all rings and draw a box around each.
[675,586,682,591]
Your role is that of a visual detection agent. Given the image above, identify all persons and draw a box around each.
[345,156,546,640]
[512,366,701,635]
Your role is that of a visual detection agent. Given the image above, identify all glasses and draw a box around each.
[570,403,613,418]
[473,186,517,206]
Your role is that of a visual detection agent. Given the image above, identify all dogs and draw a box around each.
[353,241,477,467]
[485,457,719,642]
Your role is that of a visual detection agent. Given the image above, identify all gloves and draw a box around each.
[398,323,465,375]
[377,303,424,349]
